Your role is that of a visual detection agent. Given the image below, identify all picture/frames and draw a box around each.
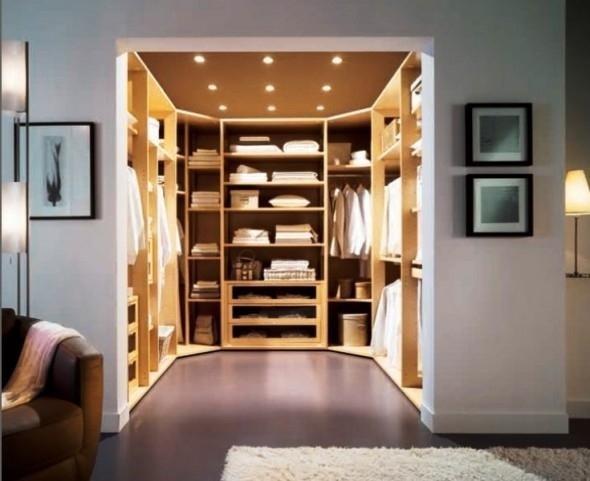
[465,172,534,238]
[464,101,534,166]
[12,120,97,221]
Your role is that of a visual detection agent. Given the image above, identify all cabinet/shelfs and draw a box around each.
[325,107,373,360]
[127,50,176,411]
[175,107,223,360]
[223,117,325,351]
[372,52,421,413]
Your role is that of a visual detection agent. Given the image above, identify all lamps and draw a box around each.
[1,179,28,315]
[0,38,36,318]
[565,168,590,277]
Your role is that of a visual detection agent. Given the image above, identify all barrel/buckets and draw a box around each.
[339,313,367,346]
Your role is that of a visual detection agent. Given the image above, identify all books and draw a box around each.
[190,279,220,299]
[187,148,220,167]
[191,242,220,257]
[190,190,220,208]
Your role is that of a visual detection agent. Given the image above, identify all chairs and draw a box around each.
[1,306,105,480]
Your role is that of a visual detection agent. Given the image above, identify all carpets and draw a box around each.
[218,443,589,481]
